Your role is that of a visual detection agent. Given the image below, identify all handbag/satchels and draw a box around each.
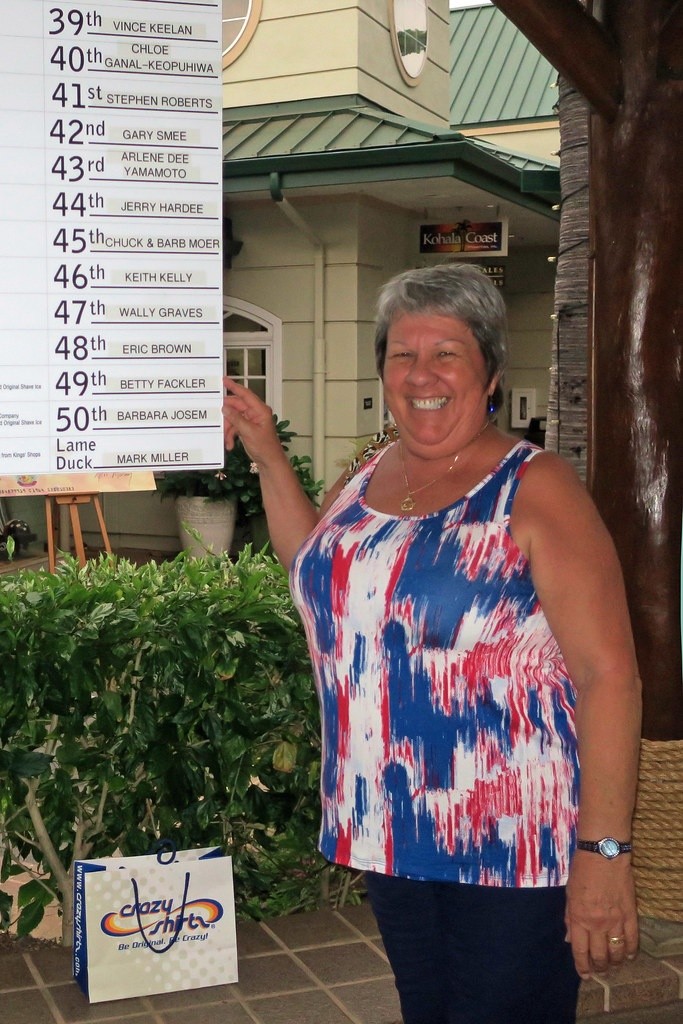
[72,842,241,1004]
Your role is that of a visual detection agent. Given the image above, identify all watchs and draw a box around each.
[577,837,632,860]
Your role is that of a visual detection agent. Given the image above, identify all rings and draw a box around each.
[609,937,624,944]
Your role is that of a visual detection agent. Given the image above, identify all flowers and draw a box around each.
[155,399,325,525]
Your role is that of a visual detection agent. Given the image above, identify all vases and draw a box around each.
[171,496,234,561]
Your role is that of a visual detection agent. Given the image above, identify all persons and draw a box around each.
[223,264,644,1024]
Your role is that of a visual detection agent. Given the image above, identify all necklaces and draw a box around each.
[399,421,490,511]
